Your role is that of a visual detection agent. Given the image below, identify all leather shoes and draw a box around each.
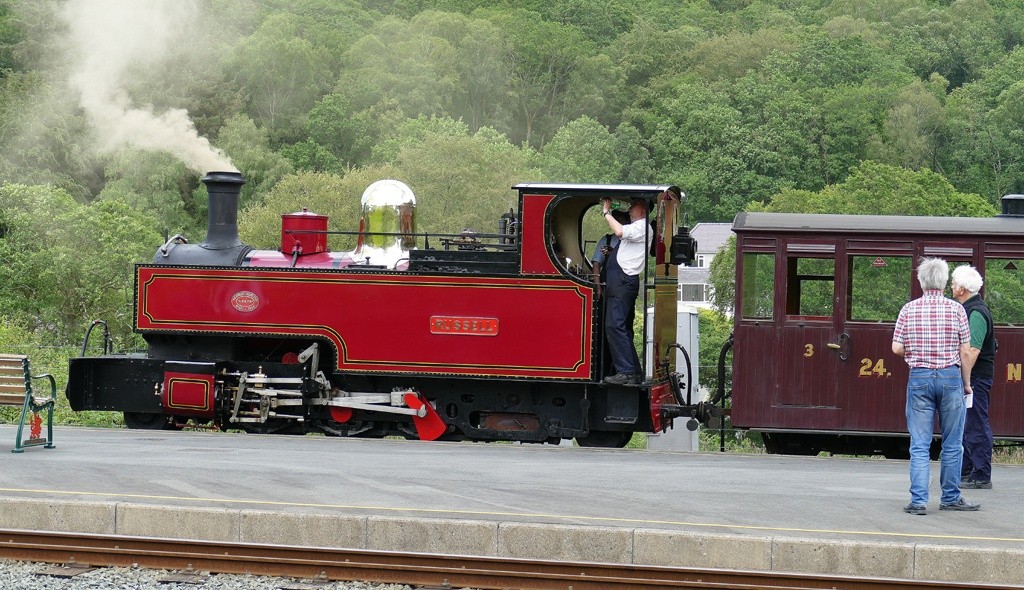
[903,502,927,515]
[940,498,980,511]
[604,373,641,384]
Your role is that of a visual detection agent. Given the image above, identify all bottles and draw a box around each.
[599,196,631,212]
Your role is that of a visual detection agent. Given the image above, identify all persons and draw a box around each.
[951,264,995,489]
[591,197,655,385]
[892,257,982,515]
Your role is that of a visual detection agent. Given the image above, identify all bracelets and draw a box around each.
[604,211,610,217]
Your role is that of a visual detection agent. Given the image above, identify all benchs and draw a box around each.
[0,353,58,453]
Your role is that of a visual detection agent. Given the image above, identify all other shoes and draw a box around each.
[959,478,993,489]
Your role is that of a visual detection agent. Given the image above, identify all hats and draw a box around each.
[631,196,655,213]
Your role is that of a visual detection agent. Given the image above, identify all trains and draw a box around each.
[64,171,1024,459]
